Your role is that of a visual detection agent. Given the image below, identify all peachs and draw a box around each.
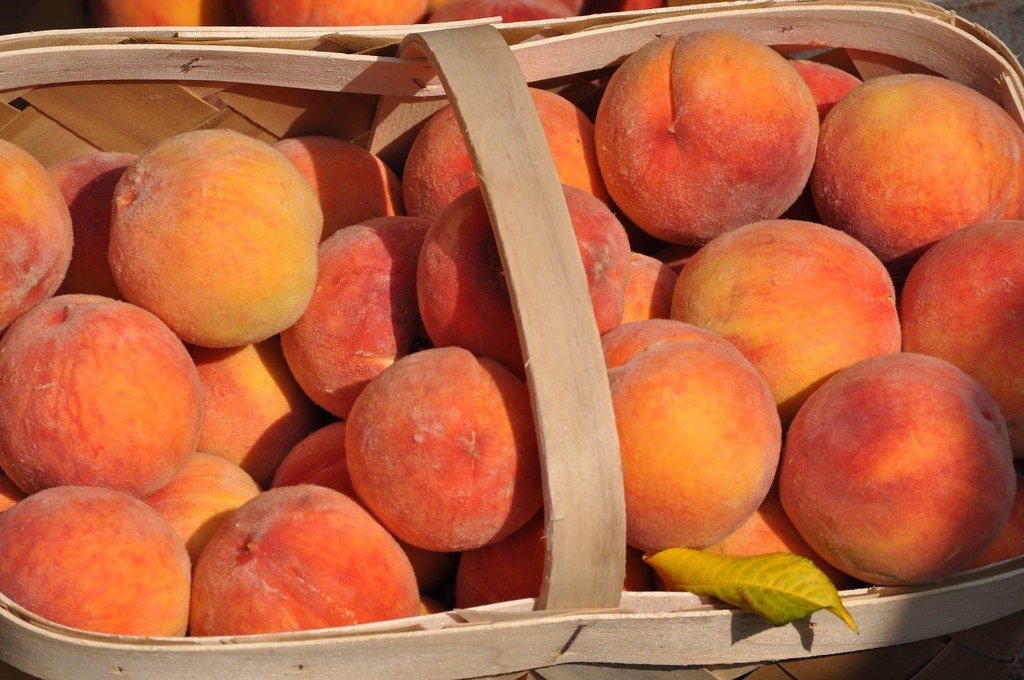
[1,0,1024,635]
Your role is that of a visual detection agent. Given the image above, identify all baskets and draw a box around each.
[0,0,1024,680]
